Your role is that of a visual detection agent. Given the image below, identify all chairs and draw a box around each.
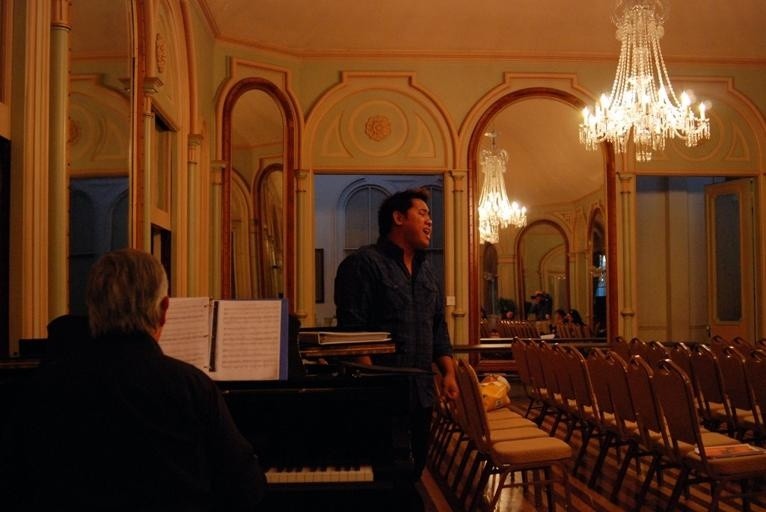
[425,336,766,512]
[479,319,592,338]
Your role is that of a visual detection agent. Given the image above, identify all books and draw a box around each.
[298,330,392,347]
[300,343,396,358]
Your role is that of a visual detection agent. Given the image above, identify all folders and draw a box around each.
[297,331,392,345]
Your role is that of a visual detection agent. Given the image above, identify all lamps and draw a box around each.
[578,0,711,161]
[477,124,528,245]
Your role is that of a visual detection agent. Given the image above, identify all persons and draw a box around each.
[335,188,460,483]
[502,291,584,329]
[3,249,266,507]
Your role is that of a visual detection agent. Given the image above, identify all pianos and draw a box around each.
[0,314,422,512]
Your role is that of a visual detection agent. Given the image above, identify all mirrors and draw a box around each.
[220,77,291,301]
[466,87,619,371]
